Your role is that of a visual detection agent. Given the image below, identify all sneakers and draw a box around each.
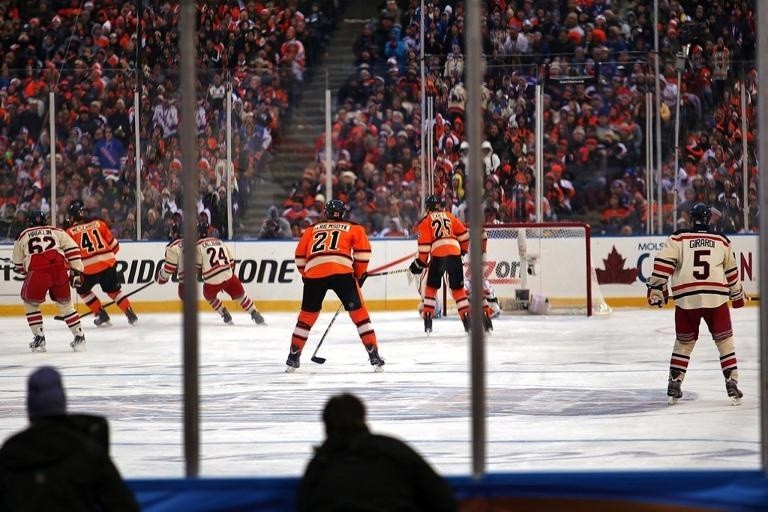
[28,328,46,348]
[726,380,743,398]
[252,311,265,324]
[462,317,471,332]
[368,350,385,366]
[69,326,85,347]
[423,317,432,332]
[127,306,138,324]
[93,309,110,325]
[223,310,232,322]
[667,377,683,398]
[286,352,300,369]
[482,312,493,332]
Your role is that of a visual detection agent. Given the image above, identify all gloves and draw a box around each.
[70,270,84,288]
[158,269,169,282]
[409,258,424,275]
[645,282,669,308]
[730,283,747,308]
[12,265,27,281]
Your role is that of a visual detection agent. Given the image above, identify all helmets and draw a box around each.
[197,219,208,238]
[324,199,346,219]
[171,223,182,234]
[425,194,442,211]
[67,200,86,225]
[689,201,712,226]
[28,208,46,226]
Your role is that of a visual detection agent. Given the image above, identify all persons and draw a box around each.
[409,0,470,195]
[0,0,138,209]
[415,230,500,318]
[138,1,330,240]
[286,199,385,372]
[385,1,409,236]
[294,394,458,512]
[197,220,265,326]
[481,0,759,230]
[409,195,470,333]
[66,199,137,325]
[648,203,745,405]
[1,366,144,511]
[158,222,185,302]
[331,1,385,232]
[12,209,86,352]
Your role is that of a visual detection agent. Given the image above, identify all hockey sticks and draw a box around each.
[311,271,368,364]
